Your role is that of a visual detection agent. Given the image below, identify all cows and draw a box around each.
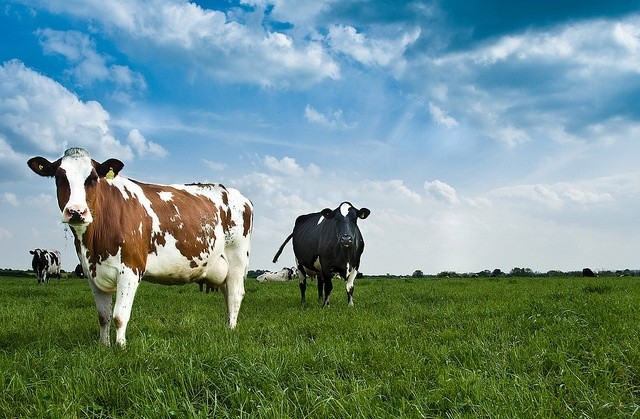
[272,201,371,310]
[26,147,254,351]
[29,248,62,285]
[256,266,299,282]
